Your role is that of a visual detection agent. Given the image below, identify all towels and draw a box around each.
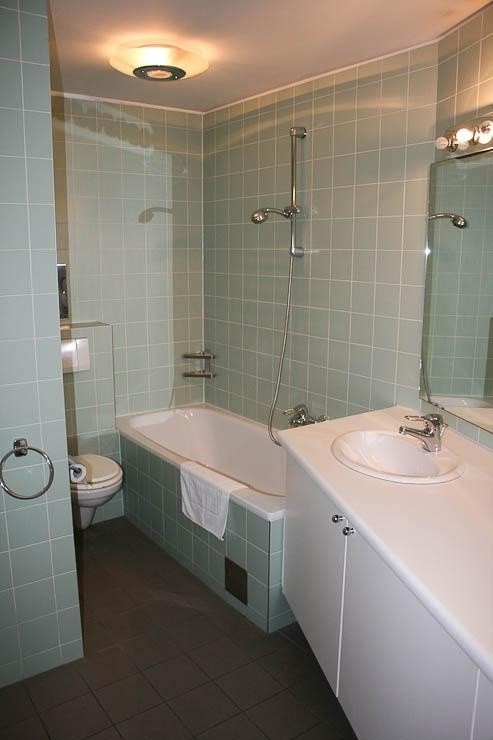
[180,459,250,542]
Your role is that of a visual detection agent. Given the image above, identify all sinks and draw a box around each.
[332,429,465,485]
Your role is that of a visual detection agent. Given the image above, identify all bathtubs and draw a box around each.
[116,403,287,522]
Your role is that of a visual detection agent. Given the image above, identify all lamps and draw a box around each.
[436,121,493,150]
[108,44,209,83]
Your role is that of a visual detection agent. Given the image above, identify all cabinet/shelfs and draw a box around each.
[278,440,491,740]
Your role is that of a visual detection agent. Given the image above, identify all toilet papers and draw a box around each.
[70,464,87,483]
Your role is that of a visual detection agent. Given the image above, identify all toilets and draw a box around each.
[69,455,124,531]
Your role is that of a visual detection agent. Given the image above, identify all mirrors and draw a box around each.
[418,152,493,438]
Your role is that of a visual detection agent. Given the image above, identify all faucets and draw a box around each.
[398,413,444,452]
[282,405,327,427]
